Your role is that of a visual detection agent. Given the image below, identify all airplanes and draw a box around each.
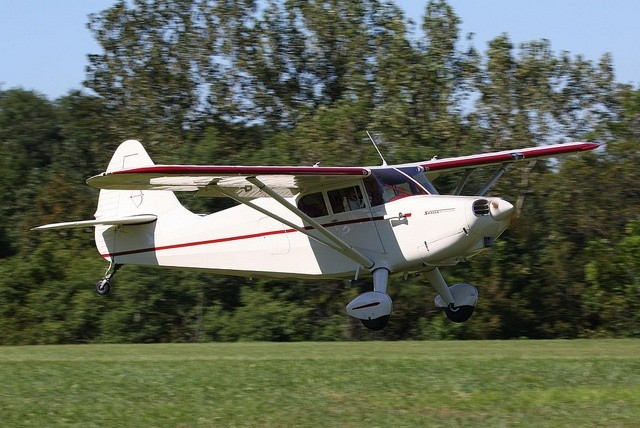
[29,129,600,329]
